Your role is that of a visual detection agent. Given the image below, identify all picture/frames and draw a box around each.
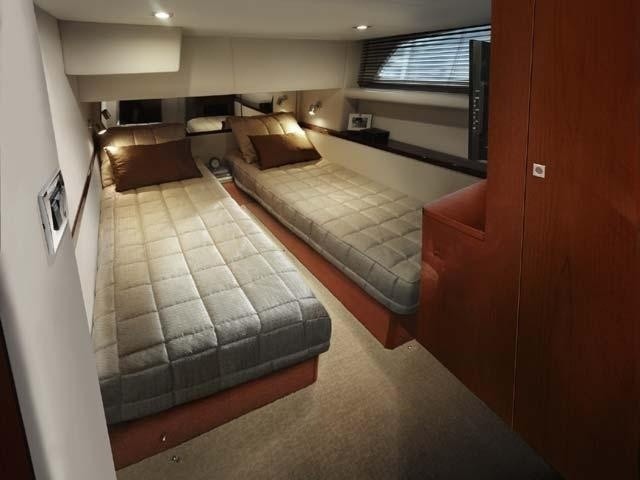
[346,113,373,132]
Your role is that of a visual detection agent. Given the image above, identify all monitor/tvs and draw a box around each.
[468,40,491,163]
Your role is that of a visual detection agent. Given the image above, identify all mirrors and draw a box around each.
[99,92,296,128]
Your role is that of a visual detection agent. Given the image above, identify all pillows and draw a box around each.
[226,111,322,171]
[248,132,323,171]
[103,137,203,193]
[98,123,185,188]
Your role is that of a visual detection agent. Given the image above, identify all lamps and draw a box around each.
[88,119,108,135]
[100,108,112,121]
[277,95,287,106]
[308,100,321,115]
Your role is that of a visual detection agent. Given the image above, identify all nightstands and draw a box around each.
[336,128,388,143]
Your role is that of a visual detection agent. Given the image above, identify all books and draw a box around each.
[206,163,234,185]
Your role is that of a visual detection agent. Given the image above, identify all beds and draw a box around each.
[91,156,332,472]
[185,114,231,132]
[223,149,430,350]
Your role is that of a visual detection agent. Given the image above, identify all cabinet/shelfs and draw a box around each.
[415,1,534,430]
